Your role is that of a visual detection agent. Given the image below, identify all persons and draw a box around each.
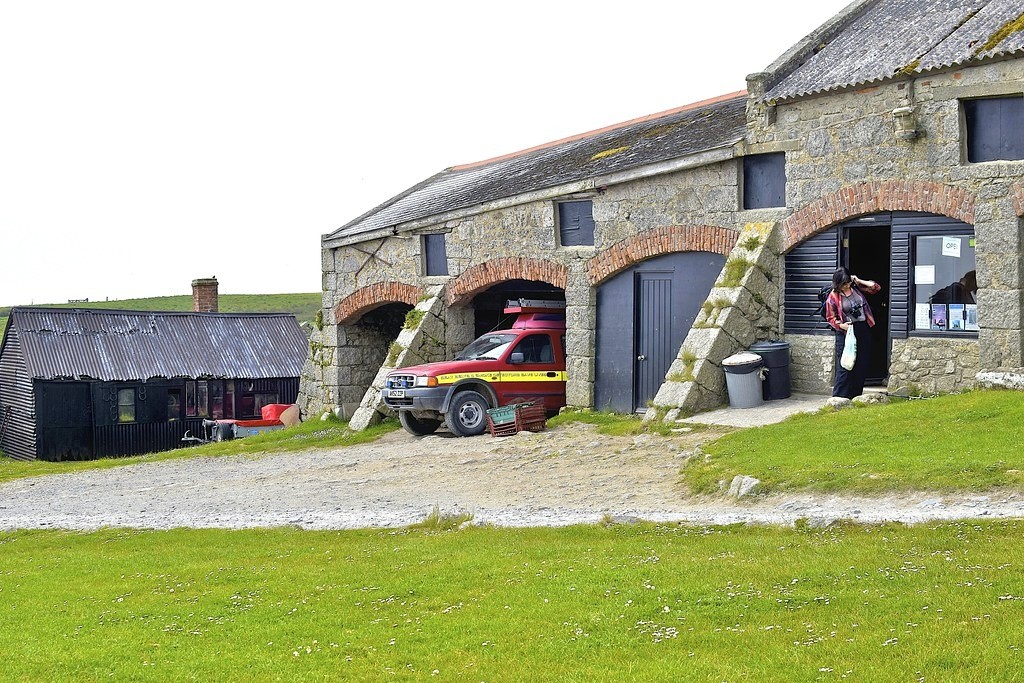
[824,266,881,398]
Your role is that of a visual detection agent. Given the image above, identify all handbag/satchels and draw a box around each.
[840,324,858,372]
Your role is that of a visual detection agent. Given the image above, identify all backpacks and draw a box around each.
[818,285,832,320]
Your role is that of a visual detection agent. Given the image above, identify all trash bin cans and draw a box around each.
[721,350,765,408]
[749,339,795,400]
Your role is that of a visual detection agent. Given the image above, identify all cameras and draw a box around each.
[850,309,861,319]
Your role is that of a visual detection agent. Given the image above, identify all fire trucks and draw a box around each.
[382,298,568,440]
